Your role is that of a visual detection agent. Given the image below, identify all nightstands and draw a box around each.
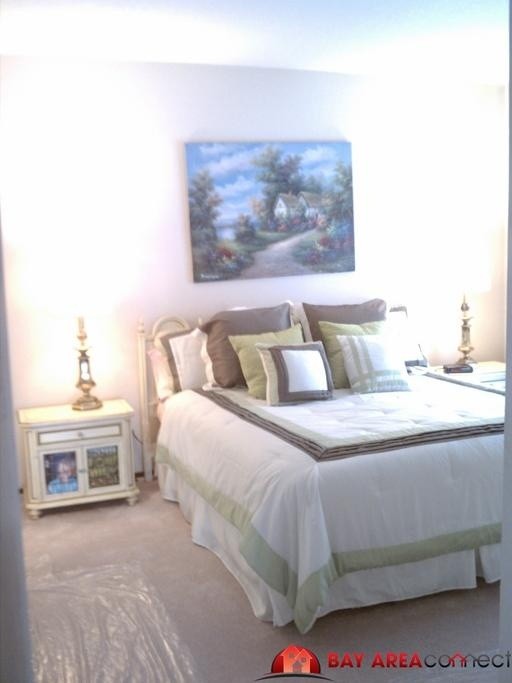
[16,398,140,521]
[427,359,506,395]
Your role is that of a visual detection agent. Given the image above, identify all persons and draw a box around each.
[46,456,77,493]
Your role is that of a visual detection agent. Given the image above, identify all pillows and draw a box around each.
[151,298,427,406]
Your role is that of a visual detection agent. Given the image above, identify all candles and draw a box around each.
[77,316,84,333]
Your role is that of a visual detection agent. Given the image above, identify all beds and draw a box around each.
[136,308,507,635]
